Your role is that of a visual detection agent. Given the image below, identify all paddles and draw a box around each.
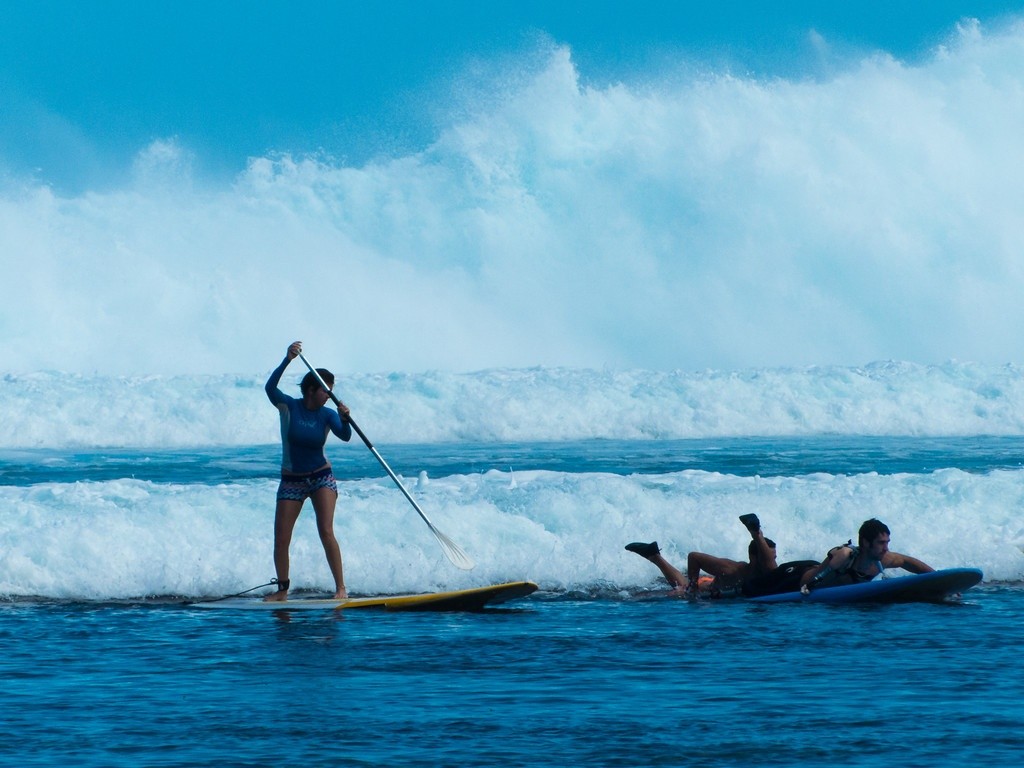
[292,341,477,571]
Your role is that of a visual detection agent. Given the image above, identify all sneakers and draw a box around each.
[739,514,761,536]
[625,541,660,559]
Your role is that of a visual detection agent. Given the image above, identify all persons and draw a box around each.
[739,513,962,598]
[262,340,352,602]
[622,536,777,599]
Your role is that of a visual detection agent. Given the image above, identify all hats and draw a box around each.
[749,537,775,552]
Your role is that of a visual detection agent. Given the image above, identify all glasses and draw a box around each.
[768,542,776,548]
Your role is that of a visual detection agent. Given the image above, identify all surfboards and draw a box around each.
[182,580,540,612]
[639,568,983,608]
